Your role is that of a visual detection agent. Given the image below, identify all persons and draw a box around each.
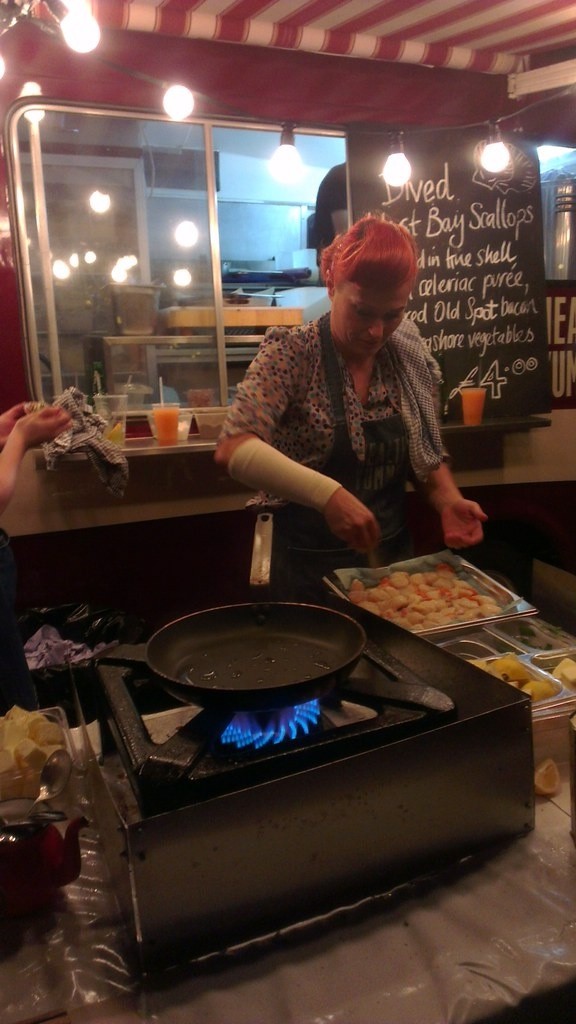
[310,158,352,265]
[212,213,489,611]
[0,396,79,718]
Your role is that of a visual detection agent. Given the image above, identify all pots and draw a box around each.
[146,601,367,714]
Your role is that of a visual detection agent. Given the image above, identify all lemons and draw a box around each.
[467,652,567,796]
[106,423,120,440]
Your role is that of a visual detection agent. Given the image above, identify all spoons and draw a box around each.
[27,749,72,813]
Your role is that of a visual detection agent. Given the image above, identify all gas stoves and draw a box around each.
[71,606,534,985]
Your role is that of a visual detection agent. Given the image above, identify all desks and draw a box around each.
[2,638,574,1022]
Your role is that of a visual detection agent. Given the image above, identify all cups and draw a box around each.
[153,403,179,441]
[94,394,127,447]
[462,388,485,425]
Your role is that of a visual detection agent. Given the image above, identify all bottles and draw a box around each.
[436,350,448,425]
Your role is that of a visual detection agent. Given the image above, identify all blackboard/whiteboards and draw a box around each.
[344,121,554,423]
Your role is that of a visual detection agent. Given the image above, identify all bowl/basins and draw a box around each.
[147,408,193,440]
[194,413,228,437]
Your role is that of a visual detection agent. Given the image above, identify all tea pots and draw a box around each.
[0,797,91,918]
